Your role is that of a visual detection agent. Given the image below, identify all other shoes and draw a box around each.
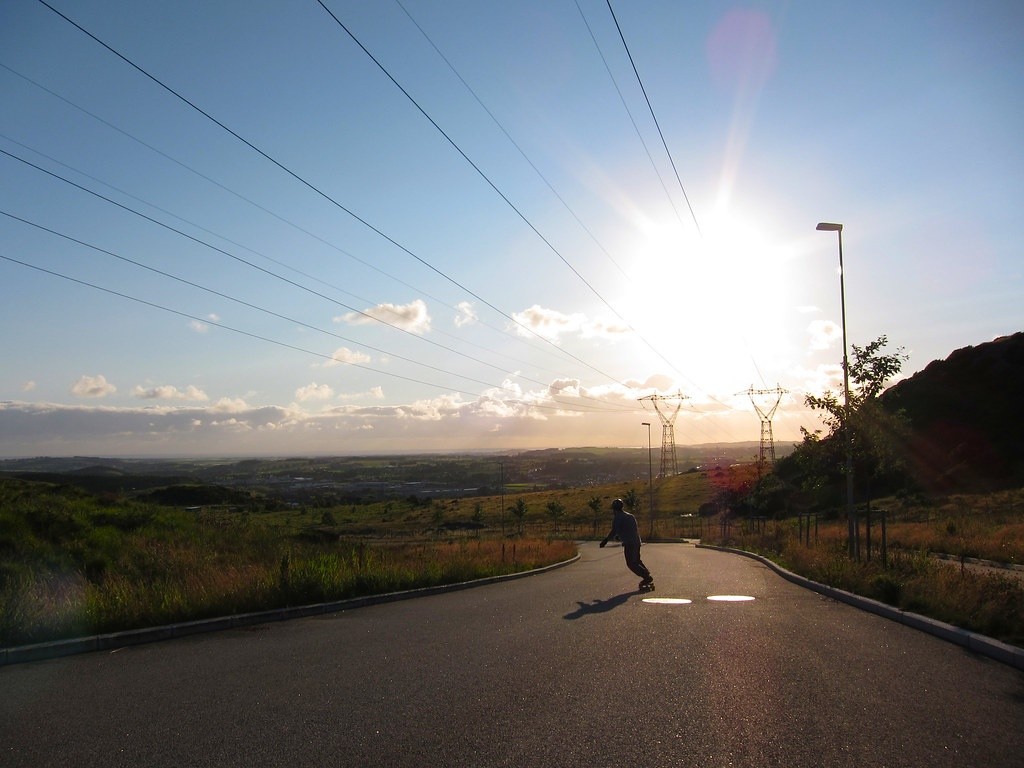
[640,576,653,586]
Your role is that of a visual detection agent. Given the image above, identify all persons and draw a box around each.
[597,497,655,593]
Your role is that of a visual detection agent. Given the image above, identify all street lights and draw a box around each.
[815,222,856,562]
[642,422,654,541]
[498,462,505,538]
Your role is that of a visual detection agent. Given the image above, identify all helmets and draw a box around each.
[611,498,624,511]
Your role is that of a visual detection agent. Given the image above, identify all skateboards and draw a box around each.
[638,581,656,592]
